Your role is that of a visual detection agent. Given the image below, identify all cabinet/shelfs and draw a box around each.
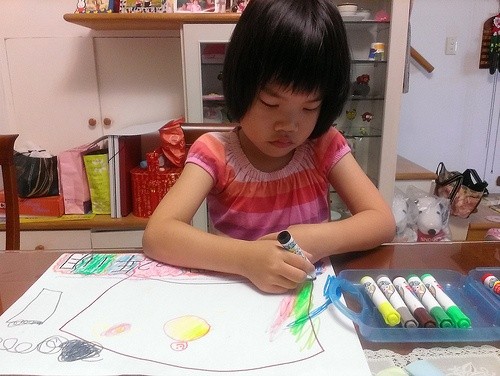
[4,30,185,157]
[0,230,144,251]
[182,0,411,235]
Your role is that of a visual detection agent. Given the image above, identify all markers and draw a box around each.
[481,273,500,295]
[360,275,472,329]
[277,231,318,280]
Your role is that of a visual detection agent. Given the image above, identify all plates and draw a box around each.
[340,15,366,22]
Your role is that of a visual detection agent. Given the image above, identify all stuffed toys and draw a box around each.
[392,195,417,243]
[415,195,453,243]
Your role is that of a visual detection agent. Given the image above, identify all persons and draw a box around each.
[141,0,396,294]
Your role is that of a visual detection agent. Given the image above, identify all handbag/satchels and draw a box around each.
[60,145,99,215]
[13,148,59,198]
[431,162,488,218]
[82,149,111,214]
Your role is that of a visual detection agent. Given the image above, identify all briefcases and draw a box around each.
[130,149,181,218]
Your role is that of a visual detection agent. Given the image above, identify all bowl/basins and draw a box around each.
[357,13,370,20]
[336,4,359,15]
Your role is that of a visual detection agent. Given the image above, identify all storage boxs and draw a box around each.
[1,191,66,217]
[129,146,183,218]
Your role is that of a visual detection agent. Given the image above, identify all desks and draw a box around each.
[0,240,500,376]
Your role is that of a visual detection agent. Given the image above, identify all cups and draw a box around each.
[368,42,386,60]
